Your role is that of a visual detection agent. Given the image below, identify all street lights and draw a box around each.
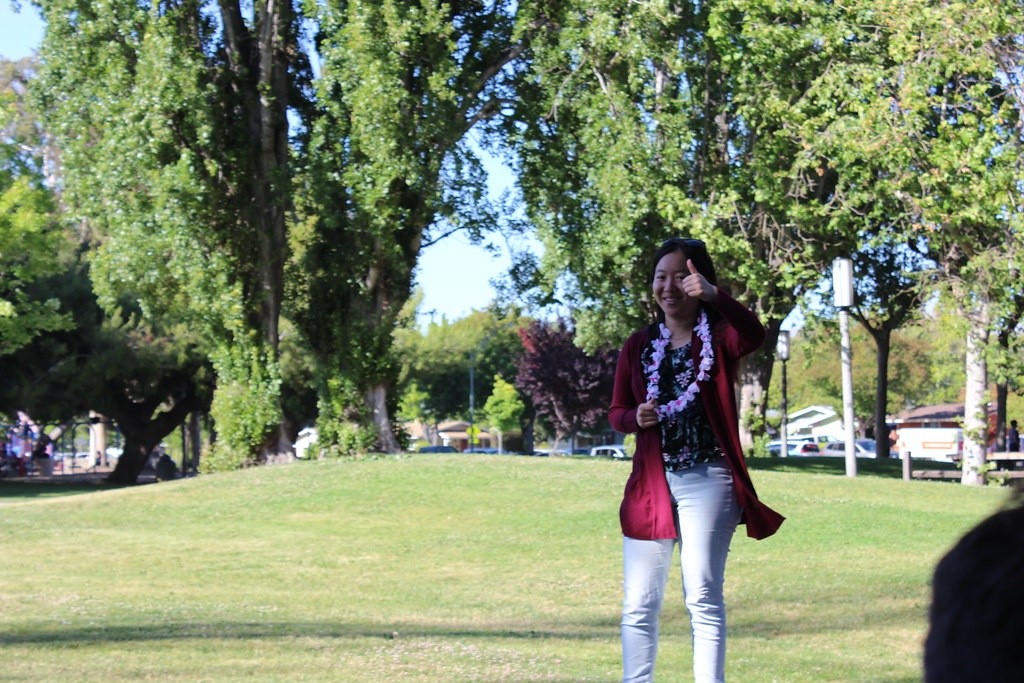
[775,329,792,458]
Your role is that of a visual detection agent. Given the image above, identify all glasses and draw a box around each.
[663,237,705,254]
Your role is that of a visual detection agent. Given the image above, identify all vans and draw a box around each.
[589,445,629,460]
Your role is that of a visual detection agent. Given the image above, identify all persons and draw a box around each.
[1008,420,1020,452]
[922,503,1024,683]
[606,239,787,681]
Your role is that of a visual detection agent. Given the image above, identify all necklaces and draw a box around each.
[645,314,713,421]
[670,332,691,342]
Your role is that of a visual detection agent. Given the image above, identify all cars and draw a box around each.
[820,437,899,459]
[419,445,459,454]
[766,436,822,458]
[534,447,590,456]
[462,446,507,455]
